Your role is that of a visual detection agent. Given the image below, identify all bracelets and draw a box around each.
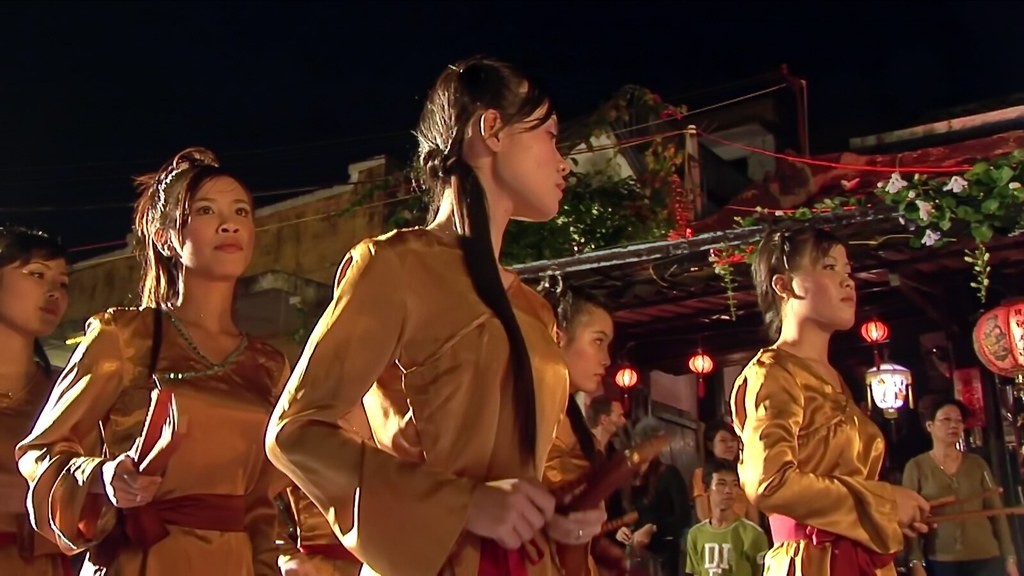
[909,560,926,566]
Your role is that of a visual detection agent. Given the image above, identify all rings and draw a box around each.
[136,494,141,502]
[576,529,583,540]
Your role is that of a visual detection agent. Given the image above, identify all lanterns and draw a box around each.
[688,348,714,397]
[615,362,639,410]
[972,295,1024,382]
[862,315,889,371]
[865,360,914,418]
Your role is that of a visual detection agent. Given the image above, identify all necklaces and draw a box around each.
[932,451,945,470]
[347,416,367,431]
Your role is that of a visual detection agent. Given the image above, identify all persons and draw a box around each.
[275,402,378,576]
[15,147,290,576]
[264,59,607,576]
[536,266,770,576]
[902,393,1019,576]
[0,227,101,576]
[730,219,939,576]
